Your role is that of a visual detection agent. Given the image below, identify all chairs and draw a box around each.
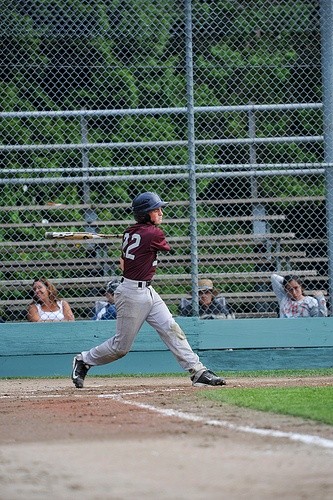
[180,298,226,309]
[95,301,108,312]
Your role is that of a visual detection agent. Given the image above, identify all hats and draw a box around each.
[107,280,121,294]
[189,279,218,297]
[132,192,169,211]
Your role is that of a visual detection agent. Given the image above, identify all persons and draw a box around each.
[179,279,235,319]
[71,192,226,388]
[27,278,74,321]
[90,279,122,320]
[271,274,328,318]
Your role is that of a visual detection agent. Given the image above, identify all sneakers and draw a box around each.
[72,354,91,388]
[191,371,227,387]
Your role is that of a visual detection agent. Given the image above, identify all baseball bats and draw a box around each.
[45,232,123,240]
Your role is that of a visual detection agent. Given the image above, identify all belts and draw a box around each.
[120,277,151,287]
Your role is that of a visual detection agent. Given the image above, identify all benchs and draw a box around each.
[0,216,330,317]
